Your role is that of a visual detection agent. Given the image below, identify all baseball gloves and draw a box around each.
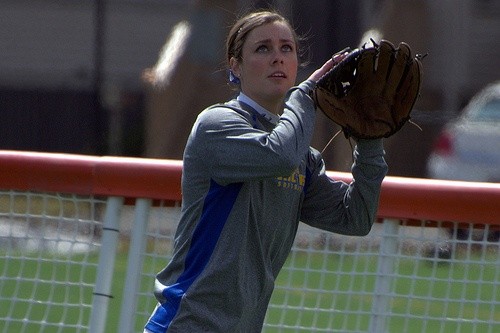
[307,36,430,143]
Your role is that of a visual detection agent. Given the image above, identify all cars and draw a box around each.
[426,84,500,240]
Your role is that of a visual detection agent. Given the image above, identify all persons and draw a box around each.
[142,10,389,333]
